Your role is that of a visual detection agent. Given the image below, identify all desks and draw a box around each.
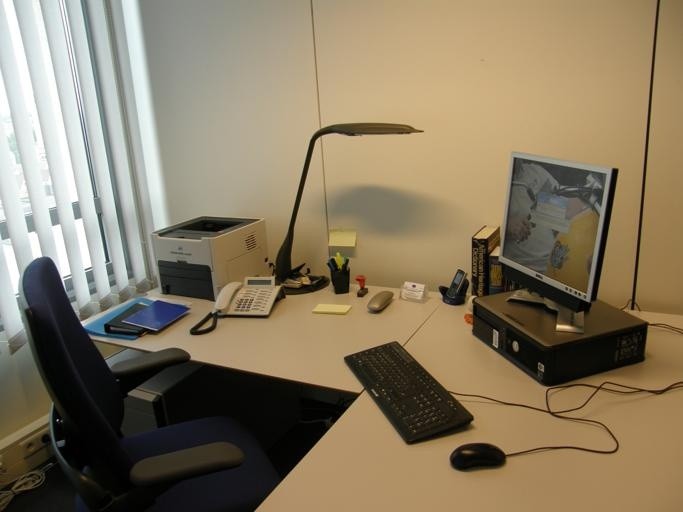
[79,280,442,395]
[255,293,683,512]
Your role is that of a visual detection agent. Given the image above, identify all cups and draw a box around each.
[330,266,350,295]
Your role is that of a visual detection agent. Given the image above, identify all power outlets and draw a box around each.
[19,425,55,469]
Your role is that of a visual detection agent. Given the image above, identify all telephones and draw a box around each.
[210,275,286,318]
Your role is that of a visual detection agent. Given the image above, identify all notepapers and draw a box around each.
[313,300,352,316]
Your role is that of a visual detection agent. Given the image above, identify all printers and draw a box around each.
[152,220,267,303]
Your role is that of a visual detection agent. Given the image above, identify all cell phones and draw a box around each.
[445,269,468,299]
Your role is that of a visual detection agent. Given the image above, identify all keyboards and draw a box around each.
[341,339,476,445]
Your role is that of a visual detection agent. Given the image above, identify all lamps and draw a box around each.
[274,123,425,298]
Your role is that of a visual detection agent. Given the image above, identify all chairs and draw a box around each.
[16,257,282,512]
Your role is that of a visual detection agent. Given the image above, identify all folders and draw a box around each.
[82,297,156,339]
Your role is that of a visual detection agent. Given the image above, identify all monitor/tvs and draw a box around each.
[497,151,619,334]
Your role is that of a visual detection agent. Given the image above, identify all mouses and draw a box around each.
[449,442,507,474]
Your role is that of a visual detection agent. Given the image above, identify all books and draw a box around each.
[103,300,192,336]
[471,224,501,298]
[486,240,514,295]
